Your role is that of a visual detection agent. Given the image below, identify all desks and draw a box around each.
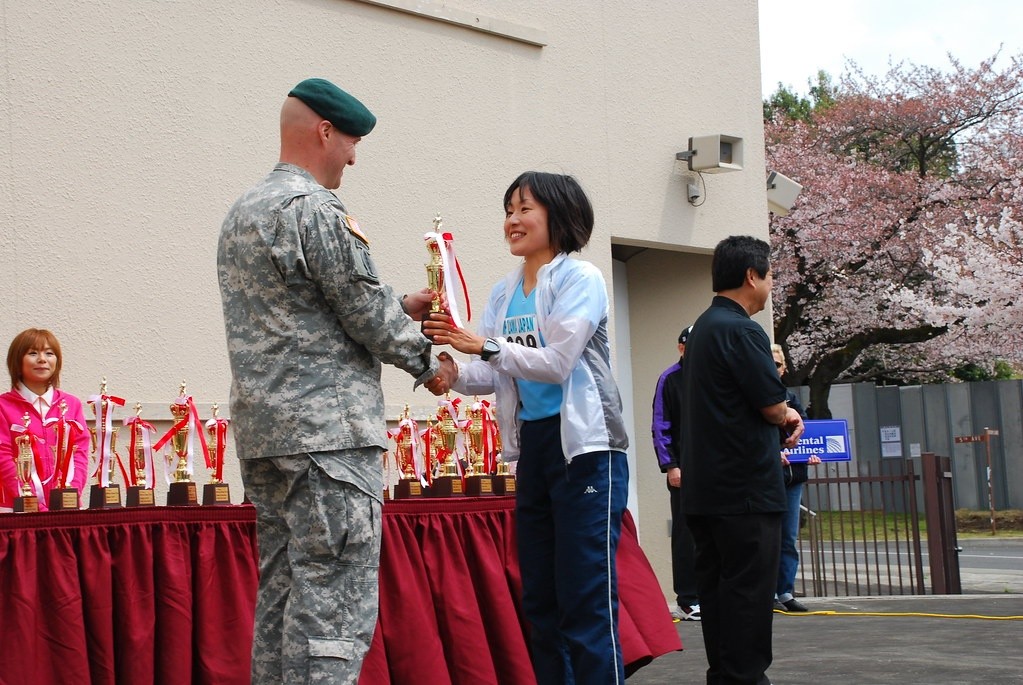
[0,488,686,685]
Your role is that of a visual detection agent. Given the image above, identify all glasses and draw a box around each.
[766,274,778,280]
[776,362,783,368]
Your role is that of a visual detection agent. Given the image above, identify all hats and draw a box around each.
[678,325,694,343]
[288,78,376,136]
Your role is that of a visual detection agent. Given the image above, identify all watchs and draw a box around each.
[481,337,500,361]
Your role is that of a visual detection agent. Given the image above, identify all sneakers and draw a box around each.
[673,605,701,621]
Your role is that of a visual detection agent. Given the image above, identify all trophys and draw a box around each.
[382,392,517,501]
[14,376,231,511]
[421,212,471,344]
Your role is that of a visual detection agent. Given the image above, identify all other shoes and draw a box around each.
[773,599,789,612]
[782,599,808,611]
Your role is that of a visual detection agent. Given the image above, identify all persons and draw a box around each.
[651,326,701,620]
[423,171,631,685]
[770,344,822,612]
[0,328,88,513]
[217,80,459,685]
[683,235,805,685]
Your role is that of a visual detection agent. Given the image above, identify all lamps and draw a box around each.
[675,133,744,207]
[766,169,804,218]
